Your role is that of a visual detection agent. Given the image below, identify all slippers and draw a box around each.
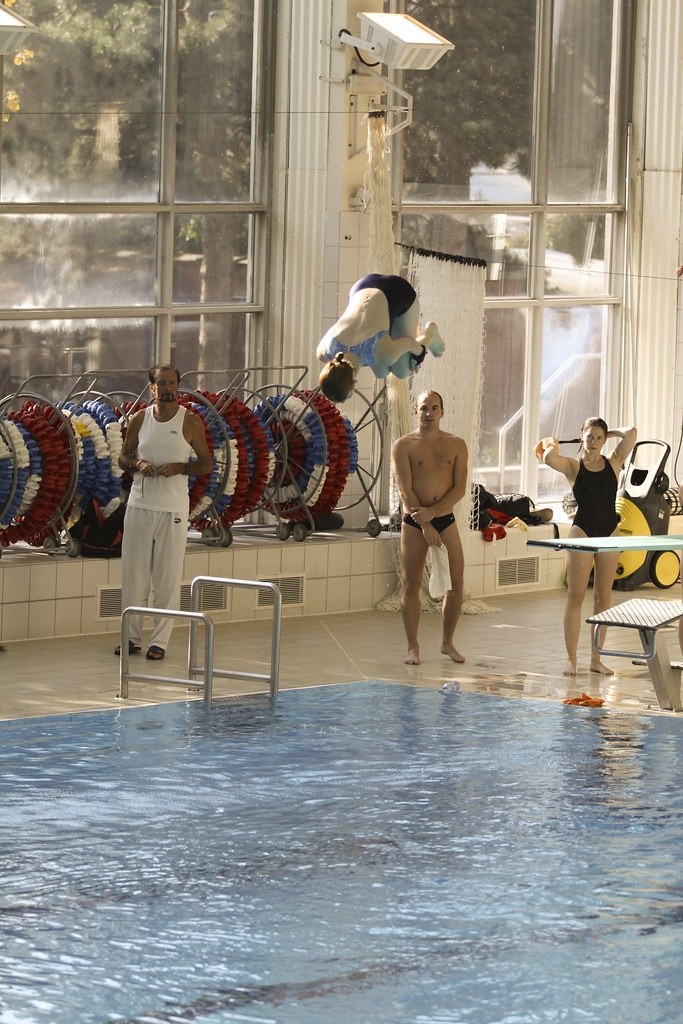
[147,647,166,660]
[115,641,142,654]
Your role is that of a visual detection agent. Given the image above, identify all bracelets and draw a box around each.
[183,462,192,476]
[136,459,146,471]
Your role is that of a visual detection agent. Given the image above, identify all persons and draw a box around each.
[115,366,214,662]
[392,390,469,666]
[315,273,445,404]
[540,417,638,677]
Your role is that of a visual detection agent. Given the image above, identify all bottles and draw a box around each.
[442,681,460,692]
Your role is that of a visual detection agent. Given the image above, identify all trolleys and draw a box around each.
[580,440,678,590]
